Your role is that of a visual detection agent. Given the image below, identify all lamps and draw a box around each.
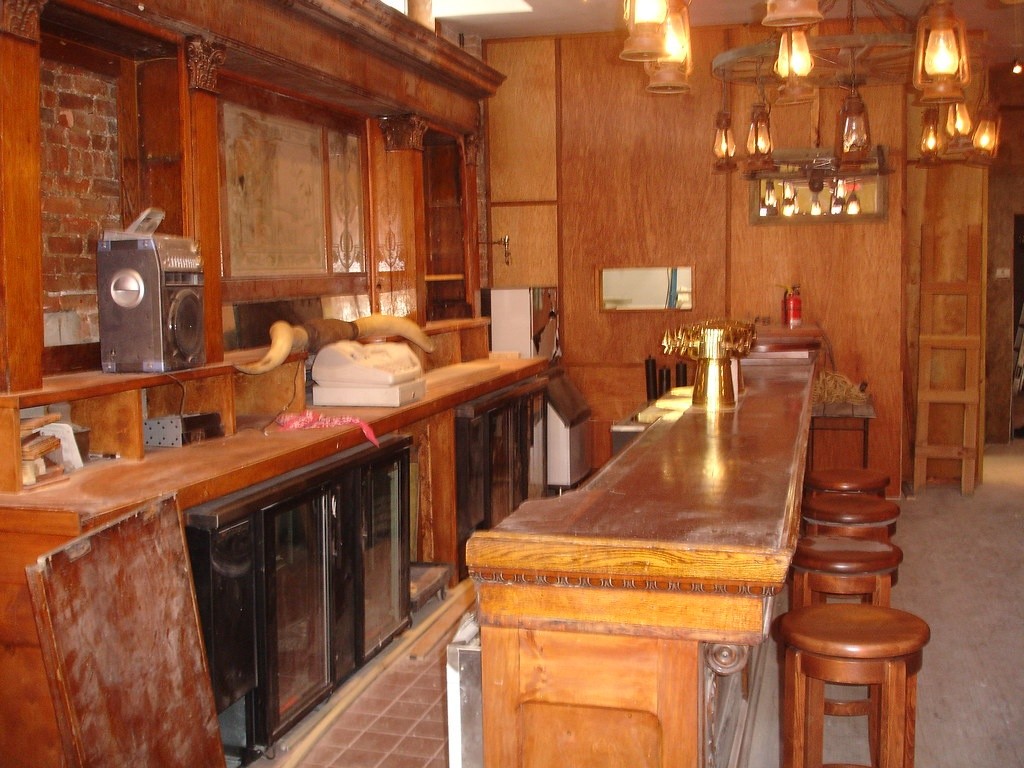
[618,0,671,62]
[760,179,864,216]
[916,102,1001,170]
[644,0,693,94]
[913,0,971,104]
[773,26,814,104]
[711,1,915,171]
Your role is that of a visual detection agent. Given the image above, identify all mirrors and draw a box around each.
[749,147,890,224]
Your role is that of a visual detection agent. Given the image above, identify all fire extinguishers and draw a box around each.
[784,284,802,326]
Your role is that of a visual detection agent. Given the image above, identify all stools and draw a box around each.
[771,473,931,767]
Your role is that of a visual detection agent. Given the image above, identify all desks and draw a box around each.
[810,394,877,471]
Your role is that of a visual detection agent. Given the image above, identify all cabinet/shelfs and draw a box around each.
[0,2,560,768]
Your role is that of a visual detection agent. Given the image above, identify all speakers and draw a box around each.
[97,240,205,374]
